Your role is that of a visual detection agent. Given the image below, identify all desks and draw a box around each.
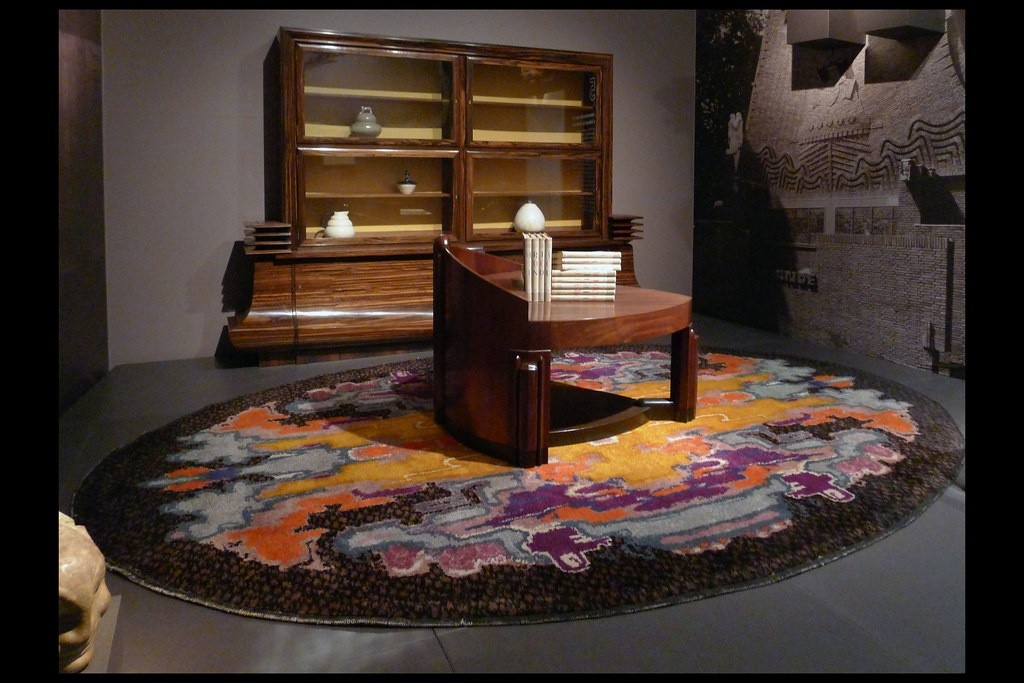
[432,235,700,471]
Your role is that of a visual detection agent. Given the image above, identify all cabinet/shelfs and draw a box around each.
[226,26,643,368]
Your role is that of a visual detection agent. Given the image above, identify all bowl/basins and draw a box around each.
[396,183,416,195]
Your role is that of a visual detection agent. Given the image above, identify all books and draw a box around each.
[522,232,622,303]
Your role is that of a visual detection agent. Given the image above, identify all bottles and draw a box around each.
[513,203,546,234]
[323,210,355,239]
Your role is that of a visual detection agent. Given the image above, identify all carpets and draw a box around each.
[70,346,965,625]
